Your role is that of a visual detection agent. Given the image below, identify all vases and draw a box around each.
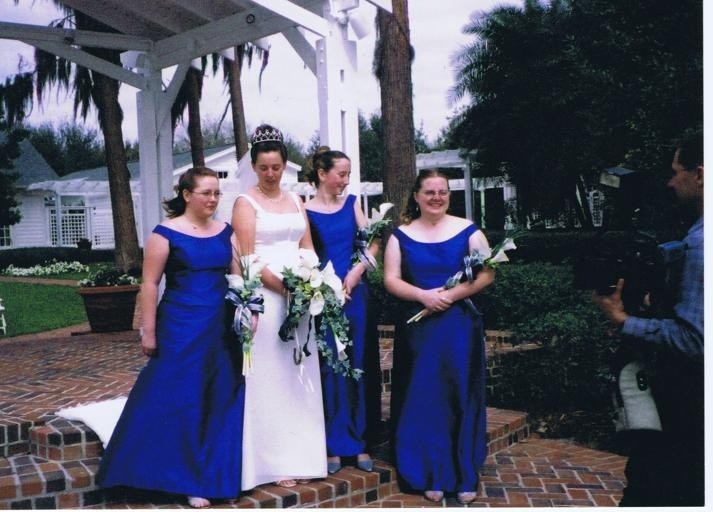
[79,285,140,333]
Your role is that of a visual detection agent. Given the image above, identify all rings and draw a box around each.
[441,298,446,302]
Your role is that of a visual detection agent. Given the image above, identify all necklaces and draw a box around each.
[255,185,284,204]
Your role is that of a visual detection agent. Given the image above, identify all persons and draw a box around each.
[384,166,498,503]
[304,145,383,474]
[230,122,328,491]
[600,125,704,506]
[94,164,243,508]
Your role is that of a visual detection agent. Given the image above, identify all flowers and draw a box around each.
[77,266,137,287]
[407,237,515,323]
[225,202,394,380]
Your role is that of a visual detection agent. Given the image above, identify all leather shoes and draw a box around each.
[458,491,476,504]
[425,489,442,503]
[355,457,374,472]
[328,460,341,475]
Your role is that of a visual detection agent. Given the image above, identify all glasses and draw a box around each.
[191,191,222,198]
[421,190,450,198]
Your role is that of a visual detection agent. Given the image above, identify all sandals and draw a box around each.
[274,480,298,488]
[188,495,212,509]
